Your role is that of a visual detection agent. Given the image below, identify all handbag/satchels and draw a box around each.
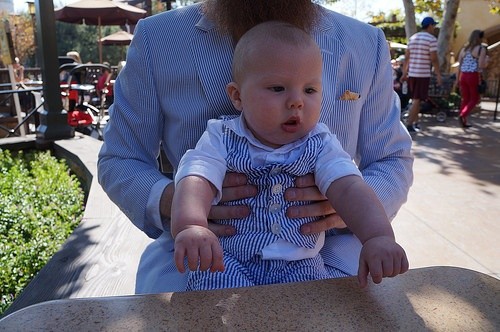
[480,80,487,94]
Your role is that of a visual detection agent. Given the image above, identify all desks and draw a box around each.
[0,266,500,332]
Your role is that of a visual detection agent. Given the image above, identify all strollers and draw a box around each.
[400,65,456,123]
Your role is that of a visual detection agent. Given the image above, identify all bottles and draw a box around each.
[402,81,408,94]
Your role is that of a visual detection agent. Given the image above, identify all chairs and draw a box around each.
[58,56,114,141]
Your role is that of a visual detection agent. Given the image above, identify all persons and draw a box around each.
[399,17,441,132]
[48,51,118,115]
[458,30,488,127]
[391,53,489,112]
[98,0,414,294]
[170,21,409,291]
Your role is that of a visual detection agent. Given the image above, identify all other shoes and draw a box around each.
[458,116,472,128]
[406,123,422,133]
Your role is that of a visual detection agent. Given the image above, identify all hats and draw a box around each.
[421,17,439,26]
